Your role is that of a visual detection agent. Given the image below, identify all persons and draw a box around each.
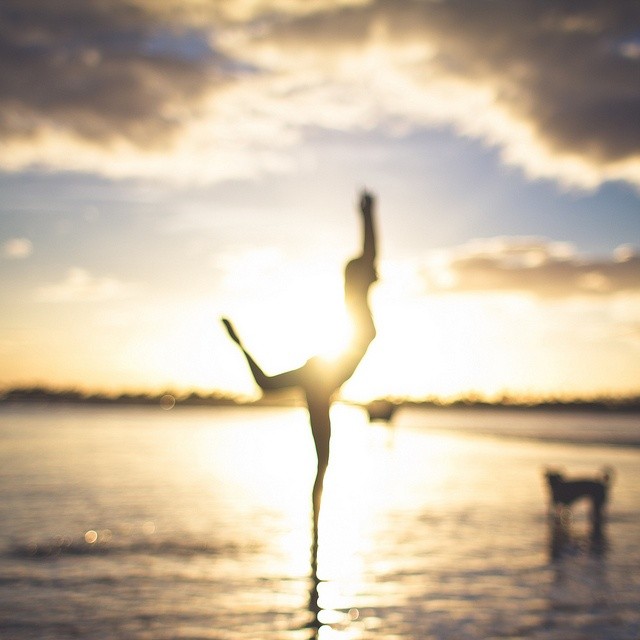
[220,186,380,524]
